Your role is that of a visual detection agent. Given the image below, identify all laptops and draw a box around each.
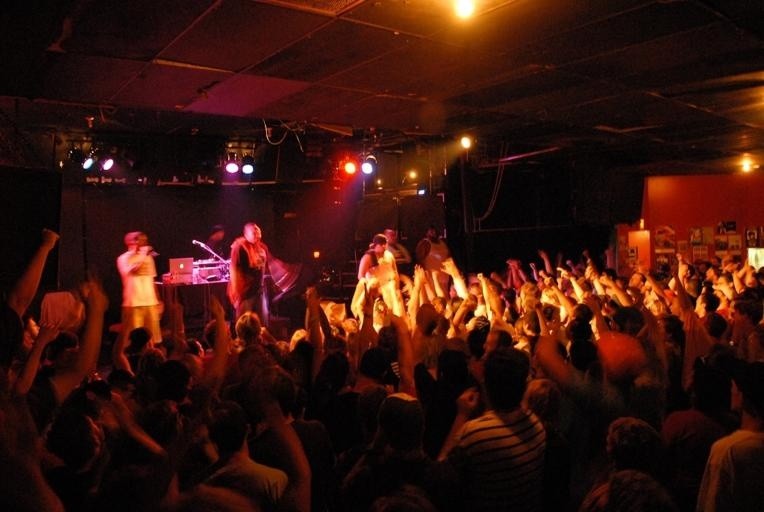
[169,257,193,274]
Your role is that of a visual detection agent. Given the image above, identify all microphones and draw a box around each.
[139,246,160,257]
[192,240,205,247]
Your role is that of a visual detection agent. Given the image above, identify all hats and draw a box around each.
[592,330,648,379]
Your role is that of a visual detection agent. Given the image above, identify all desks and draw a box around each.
[155,276,231,332]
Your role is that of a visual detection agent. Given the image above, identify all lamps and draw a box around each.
[218,139,258,175]
[76,136,124,173]
[344,140,378,174]
